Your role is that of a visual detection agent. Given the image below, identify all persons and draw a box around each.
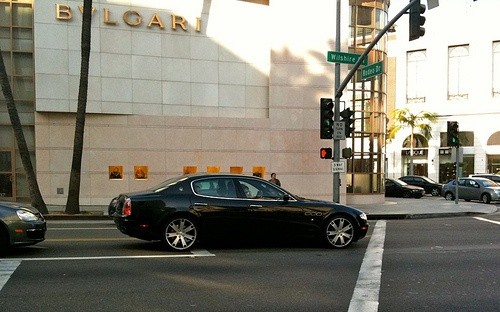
[267,173,281,187]
[241,184,264,198]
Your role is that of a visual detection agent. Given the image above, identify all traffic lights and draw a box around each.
[409,0,426,42]
[446,120,460,147]
[319,148,332,159]
[339,107,356,138]
[342,147,352,158]
[320,98,334,139]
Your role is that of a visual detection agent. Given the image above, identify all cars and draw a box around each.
[385,178,426,199]
[441,177,500,204]
[468,174,500,187]
[0,201,48,258]
[398,175,443,197]
[111,171,369,252]
